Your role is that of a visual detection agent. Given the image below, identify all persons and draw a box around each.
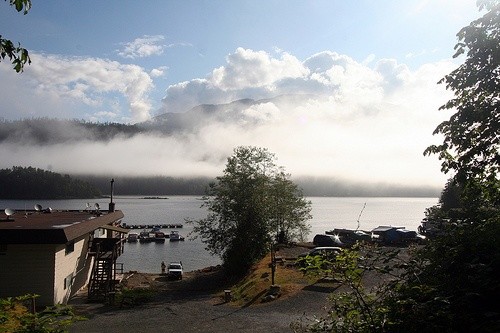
[161,260,183,273]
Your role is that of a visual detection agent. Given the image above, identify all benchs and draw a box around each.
[224,289,231,303]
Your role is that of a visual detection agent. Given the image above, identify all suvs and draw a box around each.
[167,262,183,280]
[313,234,345,249]
[297,246,345,259]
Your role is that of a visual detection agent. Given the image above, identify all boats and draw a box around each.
[126,229,181,244]
